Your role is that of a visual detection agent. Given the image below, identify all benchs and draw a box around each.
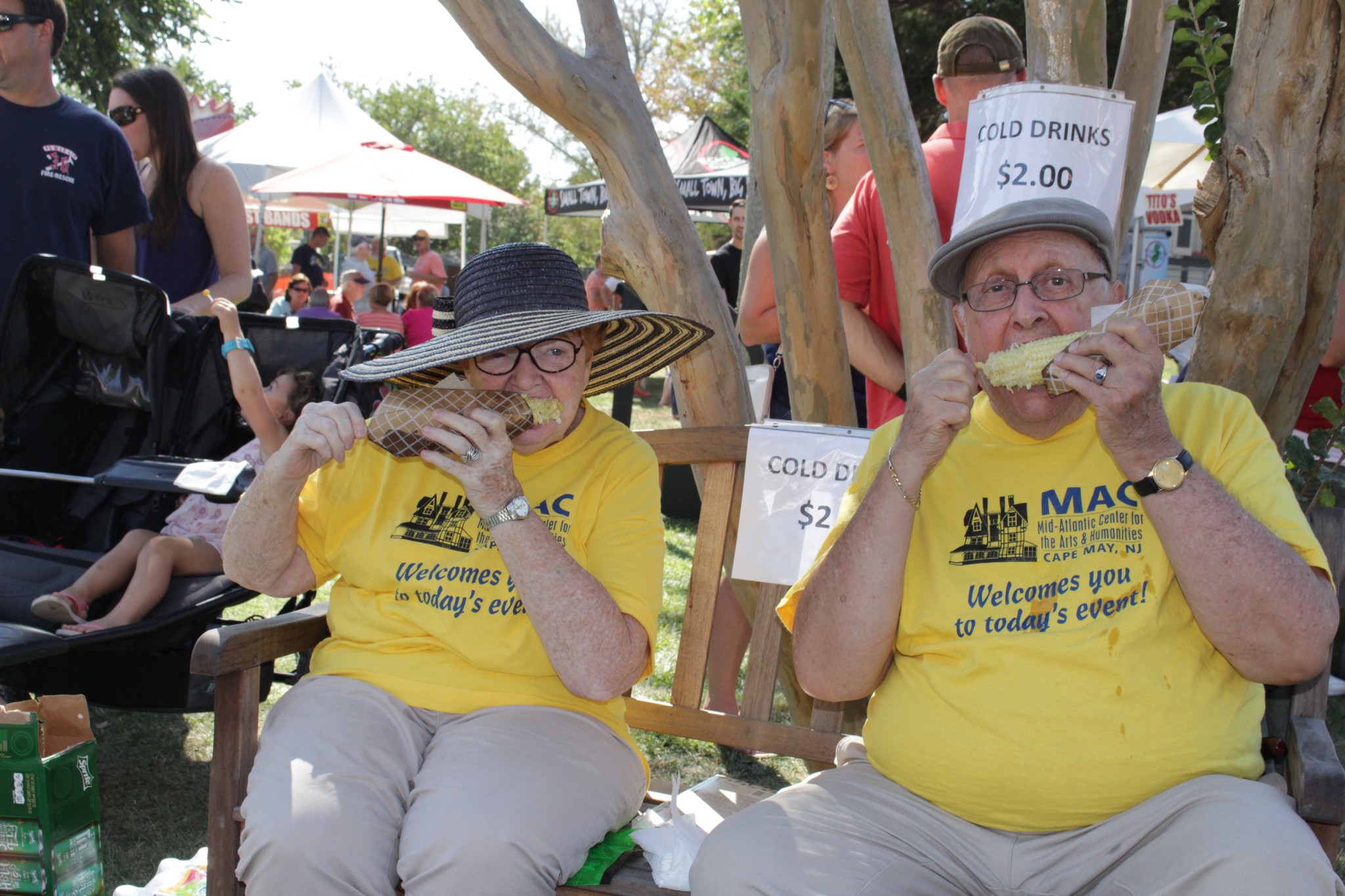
[190,421,1343,896]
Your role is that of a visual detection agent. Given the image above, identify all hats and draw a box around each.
[339,270,370,285]
[412,230,430,239]
[928,196,1116,301]
[937,14,1025,77]
[336,243,715,398]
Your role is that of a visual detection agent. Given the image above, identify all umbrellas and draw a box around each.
[241,142,530,284]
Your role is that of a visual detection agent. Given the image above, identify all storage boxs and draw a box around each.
[0,695,105,896]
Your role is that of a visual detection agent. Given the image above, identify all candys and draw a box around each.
[203,289,214,302]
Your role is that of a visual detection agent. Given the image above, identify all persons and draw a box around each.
[702,98,871,753]
[830,14,1027,429]
[1293,274,1344,460]
[220,242,716,896]
[0,0,252,322]
[250,224,447,354]
[710,199,745,309]
[688,198,1345,896]
[585,255,645,311]
[29,298,324,636]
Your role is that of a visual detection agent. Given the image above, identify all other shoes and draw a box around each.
[632,388,651,397]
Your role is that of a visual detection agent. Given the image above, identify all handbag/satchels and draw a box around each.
[744,363,774,424]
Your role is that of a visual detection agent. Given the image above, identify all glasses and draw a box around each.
[471,338,583,376]
[106,105,145,126]
[961,269,1111,312]
[414,238,425,242]
[824,98,855,127]
[293,287,310,293]
[0,12,46,32]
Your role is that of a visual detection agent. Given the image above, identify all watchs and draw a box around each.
[481,496,531,529]
[1133,449,1193,497]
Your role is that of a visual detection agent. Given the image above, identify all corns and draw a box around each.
[982,330,1088,390]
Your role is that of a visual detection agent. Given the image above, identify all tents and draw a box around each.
[544,113,749,249]
[197,73,465,272]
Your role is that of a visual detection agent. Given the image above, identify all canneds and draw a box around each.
[50,819,104,896]
[0,816,47,896]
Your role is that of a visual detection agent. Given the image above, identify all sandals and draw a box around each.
[55,622,106,637]
[31,593,89,624]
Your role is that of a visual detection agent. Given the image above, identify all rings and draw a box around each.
[459,445,479,465]
[1095,364,1109,385]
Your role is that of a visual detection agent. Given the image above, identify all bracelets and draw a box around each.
[888,446,921,509]
[896,383,906,401]
[221,337,255,361]
[607,305,611,309]
